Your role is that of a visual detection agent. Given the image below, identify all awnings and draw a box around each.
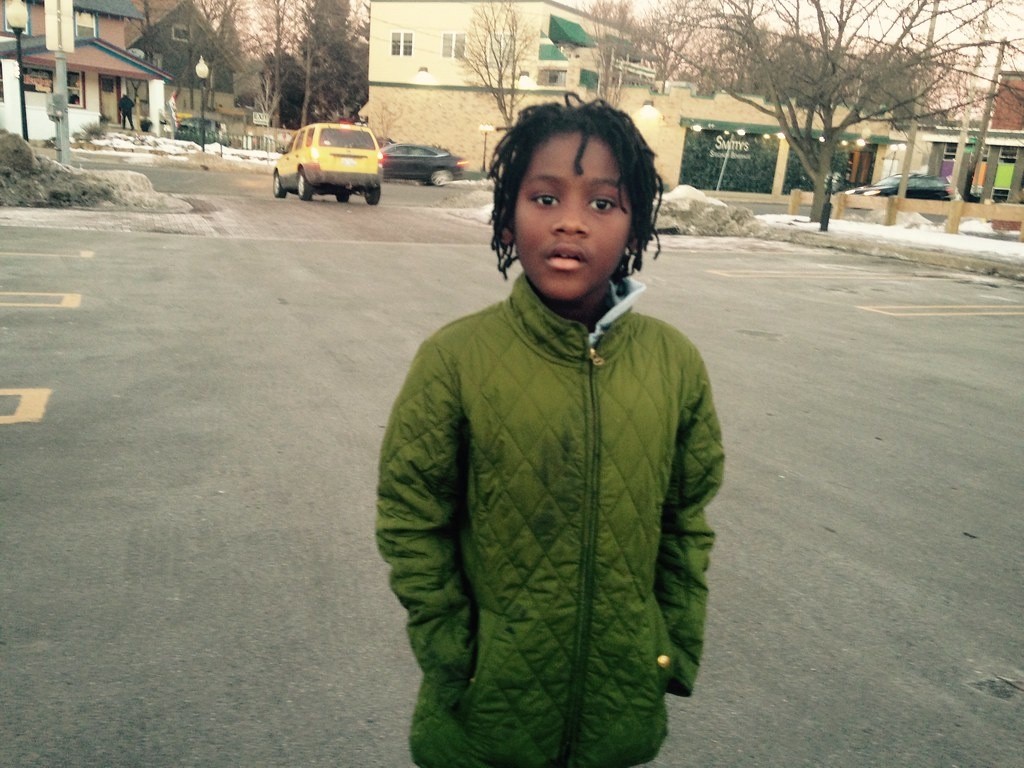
[549,14,595,48]
[606,32,663,62]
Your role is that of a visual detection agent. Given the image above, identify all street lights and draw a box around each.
[479,124,494,174]
[6,0,30,142]
[196,57,210,151]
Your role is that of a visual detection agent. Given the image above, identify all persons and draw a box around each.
[118,94,135,131]
[375,102,726,768]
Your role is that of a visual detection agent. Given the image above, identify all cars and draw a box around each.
[379,144,465,188]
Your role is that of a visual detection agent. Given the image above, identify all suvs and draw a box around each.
[844,171,953,203]
[272,123,385,205]
[179,118,229,146]
